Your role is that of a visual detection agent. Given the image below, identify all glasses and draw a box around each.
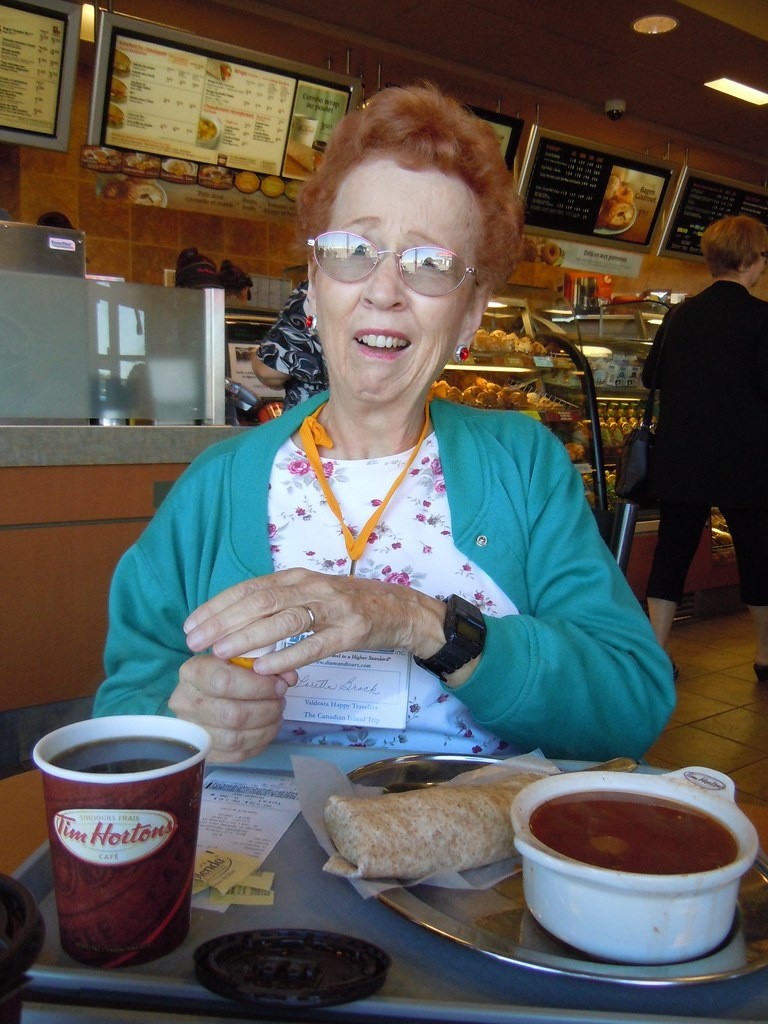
[307,231,480,297]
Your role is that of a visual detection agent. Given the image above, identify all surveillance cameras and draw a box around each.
[605,99,626,121]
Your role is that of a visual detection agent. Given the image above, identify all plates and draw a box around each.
[593,201,636,235]
[345,753,768,985]
[86,149,303,208]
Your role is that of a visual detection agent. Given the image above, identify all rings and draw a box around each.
[304,605,315,633]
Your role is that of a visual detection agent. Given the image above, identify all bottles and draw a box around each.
[583,403,657,511]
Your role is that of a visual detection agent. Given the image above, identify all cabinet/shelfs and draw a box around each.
[426,304,737,567]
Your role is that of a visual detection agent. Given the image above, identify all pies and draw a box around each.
[286,139,325,174]
[321,774,551,879]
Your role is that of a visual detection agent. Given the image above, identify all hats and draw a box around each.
[176,262,223,289]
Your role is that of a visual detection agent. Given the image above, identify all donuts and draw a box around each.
[595,175,634,231]
[542,241,560,264]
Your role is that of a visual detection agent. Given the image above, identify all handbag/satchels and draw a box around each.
[614,427,664,508]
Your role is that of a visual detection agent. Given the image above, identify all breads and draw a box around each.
[519,237,538,262]
[710,510,733,544]
[429,328,623,511]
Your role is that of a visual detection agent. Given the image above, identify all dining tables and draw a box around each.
[0,768,768,882]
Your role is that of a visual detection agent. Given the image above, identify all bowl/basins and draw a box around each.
[511,765,759,964]
[197,112,223,148]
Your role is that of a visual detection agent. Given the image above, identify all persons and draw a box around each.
[252,277,331,417]
[417,257,441,292]
[348,243,372,280]
[94,83,676,764]
[642,215,768,687]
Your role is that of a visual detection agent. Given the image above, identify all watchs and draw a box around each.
[414,594,488,683]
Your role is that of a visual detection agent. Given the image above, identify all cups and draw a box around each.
[218,154,227,166]
[33,715,213,966]
[292,114,318,148]
[0,874,44,1024]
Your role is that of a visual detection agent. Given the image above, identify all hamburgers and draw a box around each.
[105,48,131,125]
